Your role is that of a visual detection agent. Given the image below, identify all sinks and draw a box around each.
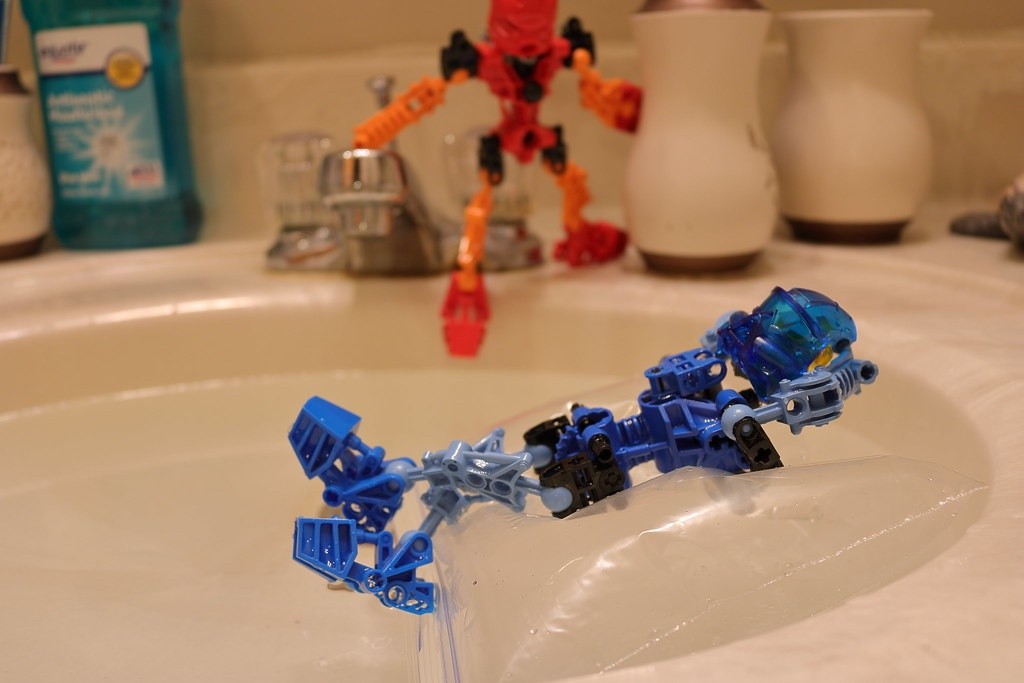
[0,229,1024,682]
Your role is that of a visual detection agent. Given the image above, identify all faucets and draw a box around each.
[317,141,433,273]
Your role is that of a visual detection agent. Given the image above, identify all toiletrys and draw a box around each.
[20,0,206,248]
